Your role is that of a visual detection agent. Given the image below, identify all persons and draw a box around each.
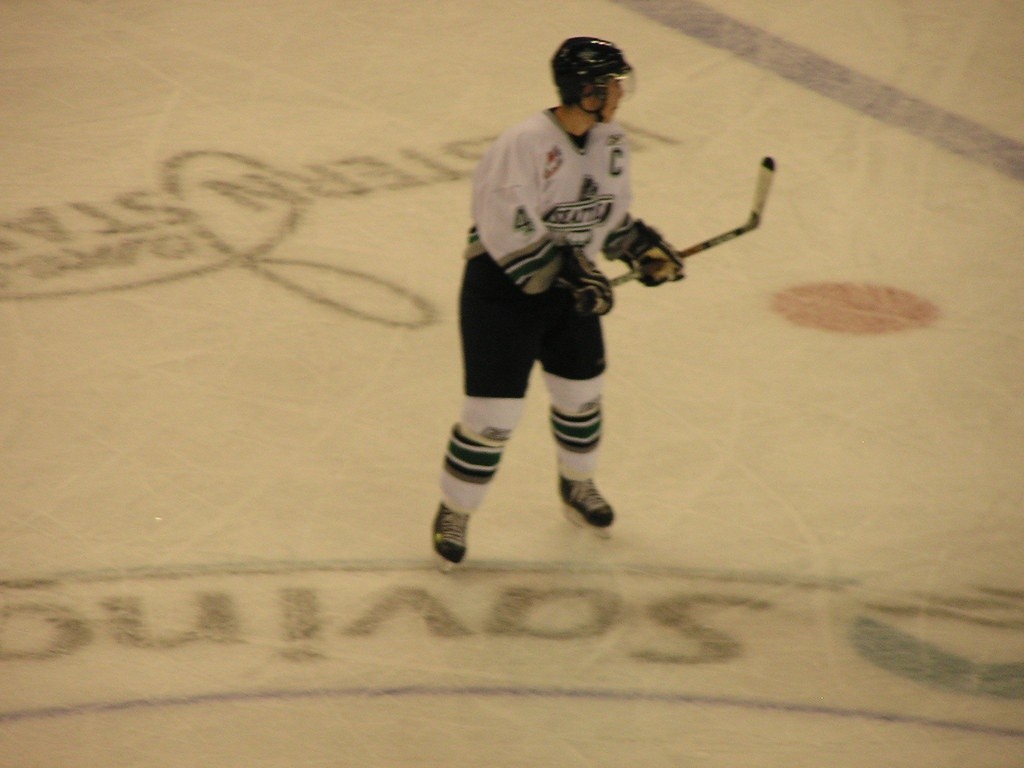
[430,37,685,564]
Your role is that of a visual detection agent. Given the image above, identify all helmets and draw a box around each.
[551,37,633,104]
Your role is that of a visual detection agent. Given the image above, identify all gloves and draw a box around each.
[546,247,613,316]
[603,218,684,286]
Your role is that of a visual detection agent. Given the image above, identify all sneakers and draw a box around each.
[560,477,613,538]
[432,503,470,573]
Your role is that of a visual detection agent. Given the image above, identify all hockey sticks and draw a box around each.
[607,156,774,287]
